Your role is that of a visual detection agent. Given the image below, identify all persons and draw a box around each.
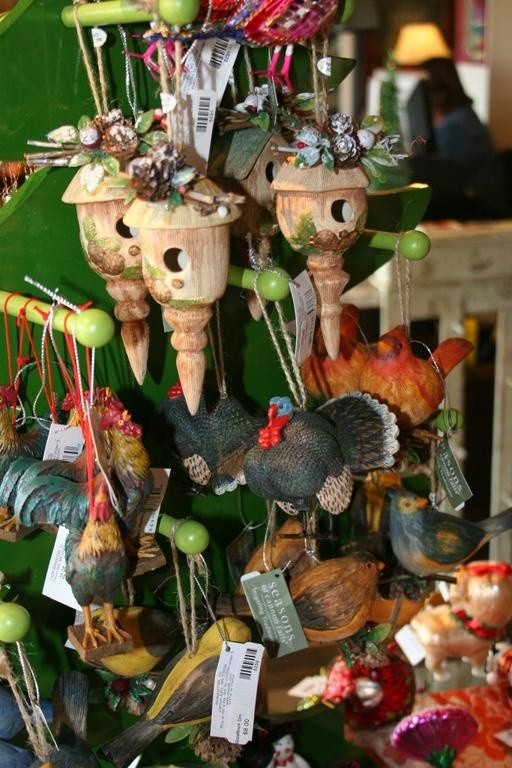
[406,58,512,222]
